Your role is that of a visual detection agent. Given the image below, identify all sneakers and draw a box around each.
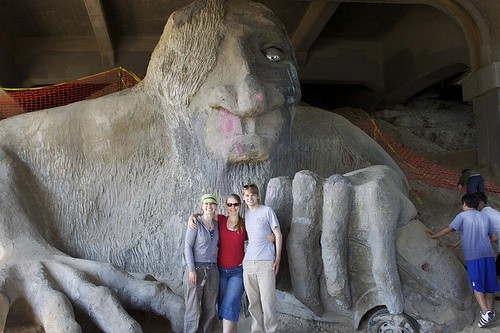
[477,310,494,328]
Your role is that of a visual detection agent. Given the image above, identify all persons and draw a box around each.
[425,192,500,328]
[188,194,275,333]
[457,168,485,193]
[241,183,283,333]
[182,194,220,333]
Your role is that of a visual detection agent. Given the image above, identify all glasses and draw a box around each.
[226,202,240,207]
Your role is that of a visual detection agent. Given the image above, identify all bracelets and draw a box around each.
[276,257,281,260]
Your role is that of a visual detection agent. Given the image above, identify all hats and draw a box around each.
[200,193,218,205]
